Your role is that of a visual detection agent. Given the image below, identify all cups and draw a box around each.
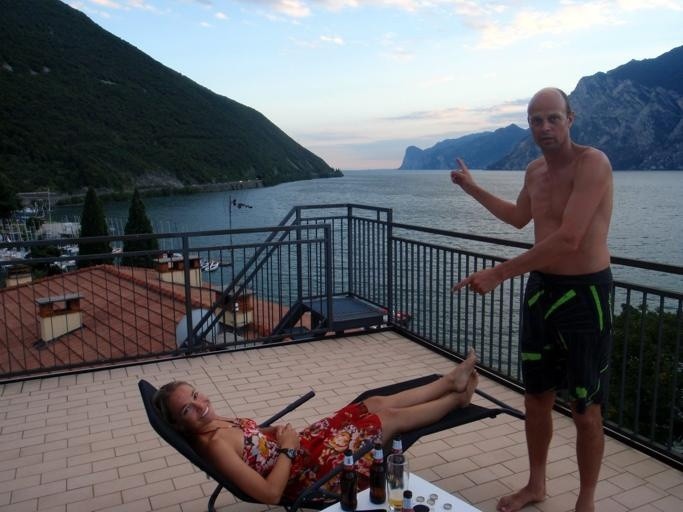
[386,453,409,509]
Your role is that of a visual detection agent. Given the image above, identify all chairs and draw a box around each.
[137,374,526,512]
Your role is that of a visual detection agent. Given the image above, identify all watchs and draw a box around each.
[278,447,295,458]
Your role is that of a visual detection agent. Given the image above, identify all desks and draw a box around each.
[320,472,484,512]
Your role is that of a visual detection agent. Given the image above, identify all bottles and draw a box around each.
[389,439,405,489]
[340,448,357,511]
[400,491,416,512]
[369,444,386,504]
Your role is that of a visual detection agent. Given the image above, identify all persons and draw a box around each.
[446,88,613,512]
[152,348,478,505]
